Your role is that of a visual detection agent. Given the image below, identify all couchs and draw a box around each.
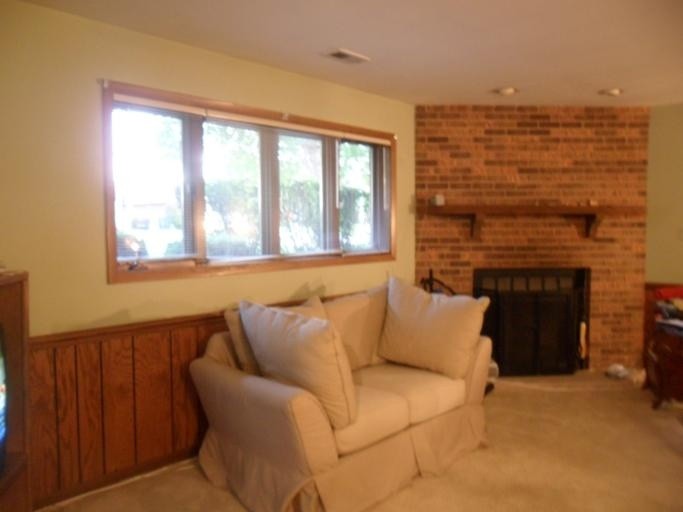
[189,282,493,511]
